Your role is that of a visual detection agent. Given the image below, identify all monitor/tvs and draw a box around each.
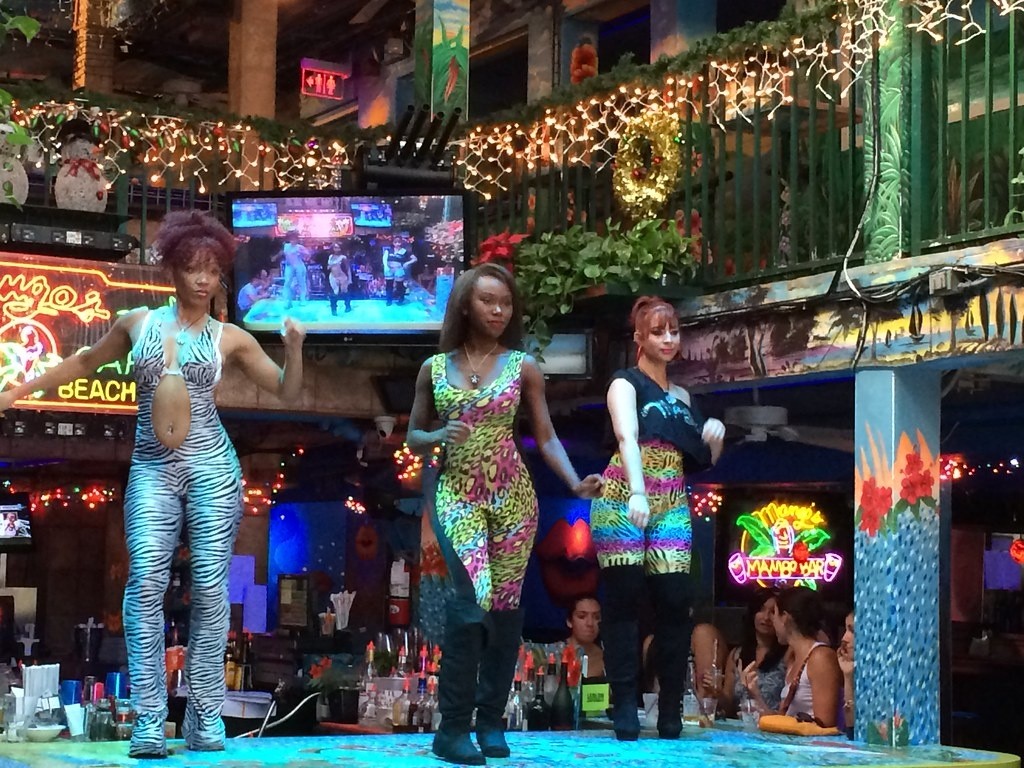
[224,188,479,346]
[0,492,37,555]
[534,329,592,381]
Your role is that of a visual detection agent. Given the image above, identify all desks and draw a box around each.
[319,721,393,734]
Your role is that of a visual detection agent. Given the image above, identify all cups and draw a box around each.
[318,614,335,634]
[643,694,658,724]
[699,698,717,728]
[5,695,25,743]
[742,698,759,723]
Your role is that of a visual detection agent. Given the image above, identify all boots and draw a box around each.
[433,612,486,765]
[476,609,525,758]
[596,564,648,740]
[649,572,690,738]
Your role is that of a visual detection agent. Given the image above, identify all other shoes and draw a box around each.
[130,716,166,757]
[181,711,226,750]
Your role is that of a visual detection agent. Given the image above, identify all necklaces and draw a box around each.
[645,370,678,404]
[172,303,206,345]
[462,340,499,385]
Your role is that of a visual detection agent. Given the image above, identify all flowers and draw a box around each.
[469,232,531,273]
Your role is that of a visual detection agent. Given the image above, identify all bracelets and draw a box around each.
[841,699,854,709]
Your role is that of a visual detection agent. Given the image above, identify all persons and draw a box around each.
[402,261,608,766]
[234,209,465,311]
[588,291,727,745]
[553,585,862,743]
[0,210,305,758]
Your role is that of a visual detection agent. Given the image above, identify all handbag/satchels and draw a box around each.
[758,712,839,735]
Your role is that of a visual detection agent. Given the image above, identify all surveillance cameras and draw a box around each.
[374,416,397,440]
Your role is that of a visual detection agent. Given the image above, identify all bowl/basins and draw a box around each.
[24,725,66,742]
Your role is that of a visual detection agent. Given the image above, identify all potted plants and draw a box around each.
[512,215,700,365]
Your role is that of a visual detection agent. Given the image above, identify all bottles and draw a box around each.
[507,644,581,731]
[224,631,254,691]
[61,671,133,742]
[359,623,442,733]
[711,638,723,694]
[683,654,700,724]
